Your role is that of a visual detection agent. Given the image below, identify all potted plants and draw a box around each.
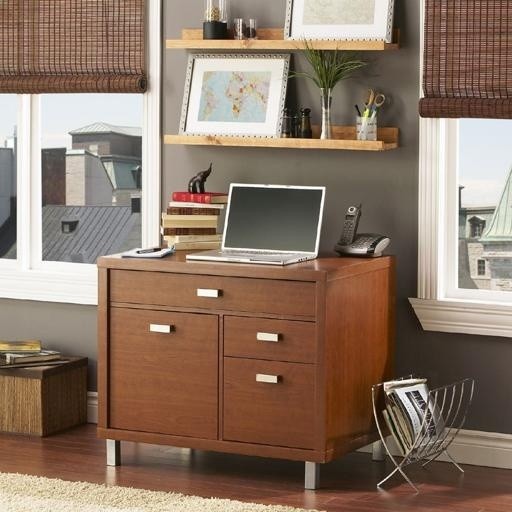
[286,35,367,140]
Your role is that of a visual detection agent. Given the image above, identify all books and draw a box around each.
[380,375,447,459]
[163,233,224,243]
[1,356,72,369]
[0,338,42,355]
[119,246,176,258]
[162,227,218,237]
[165,240,222,250]
[167,200,226,210]
[172,191,230,205]
[165,206,222,216]
[0,350,63,365]
[160,212,218,221]
[161,220,219,229]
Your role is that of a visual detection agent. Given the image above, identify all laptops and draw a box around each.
[185,182,327,266]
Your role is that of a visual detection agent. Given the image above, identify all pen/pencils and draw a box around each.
[136,248,161,254]
[354,104,377,117]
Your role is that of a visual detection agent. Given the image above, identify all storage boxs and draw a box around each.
[2,350,87,439]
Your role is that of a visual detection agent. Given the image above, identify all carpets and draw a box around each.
[1,466,328,512]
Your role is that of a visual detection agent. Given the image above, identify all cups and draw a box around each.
[233,18,257,40]
[355,116,378,141]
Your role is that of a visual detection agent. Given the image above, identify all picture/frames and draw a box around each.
[177,52,292,137]
[284,0,396,44]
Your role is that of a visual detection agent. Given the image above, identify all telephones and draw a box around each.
[334,202,392,258]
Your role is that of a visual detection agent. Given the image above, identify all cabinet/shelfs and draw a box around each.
[162,21,403,153]
[91,249,396,491]
